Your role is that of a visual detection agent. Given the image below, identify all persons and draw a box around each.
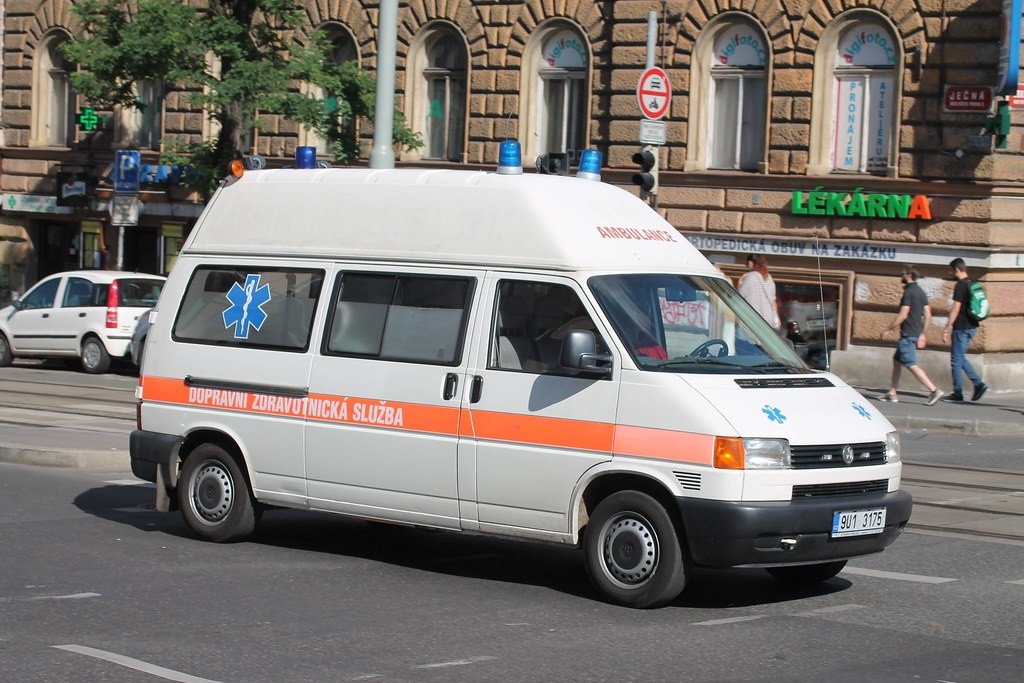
[939,257,989,402]
[613,289,668,361]
[875,265,945,407]
[736,253,783,361]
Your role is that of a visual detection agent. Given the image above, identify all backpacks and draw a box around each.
[960,279,990,321]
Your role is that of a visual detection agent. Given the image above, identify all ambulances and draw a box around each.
[129,139,914,609]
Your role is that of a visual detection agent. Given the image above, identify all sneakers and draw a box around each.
[925,390,944,406]
[972,383,989,402]
[940,392,963,403]
[879,393,899,403]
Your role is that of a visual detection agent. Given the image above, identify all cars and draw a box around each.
[0,271,169,374]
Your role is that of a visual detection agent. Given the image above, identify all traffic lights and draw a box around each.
[631,146,660,197]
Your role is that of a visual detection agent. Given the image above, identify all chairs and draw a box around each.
[493,294,600,375]
